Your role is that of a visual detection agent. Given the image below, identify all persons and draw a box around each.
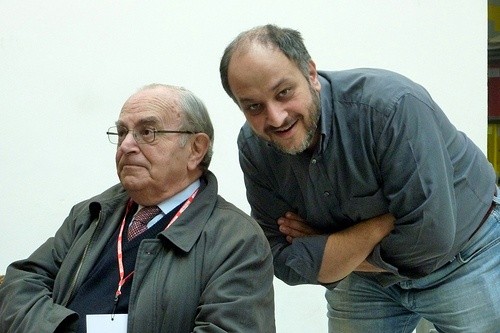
[219,23,500,333]
[0,81,277,333]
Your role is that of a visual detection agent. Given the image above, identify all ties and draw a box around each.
[128,206,162,242]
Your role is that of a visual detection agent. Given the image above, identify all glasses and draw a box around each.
[107,125,209,144]
